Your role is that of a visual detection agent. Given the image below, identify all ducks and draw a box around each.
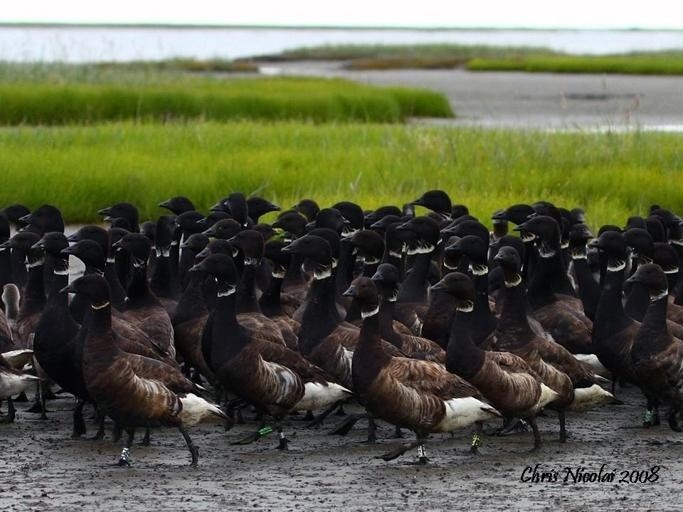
[0,188,683,469]
